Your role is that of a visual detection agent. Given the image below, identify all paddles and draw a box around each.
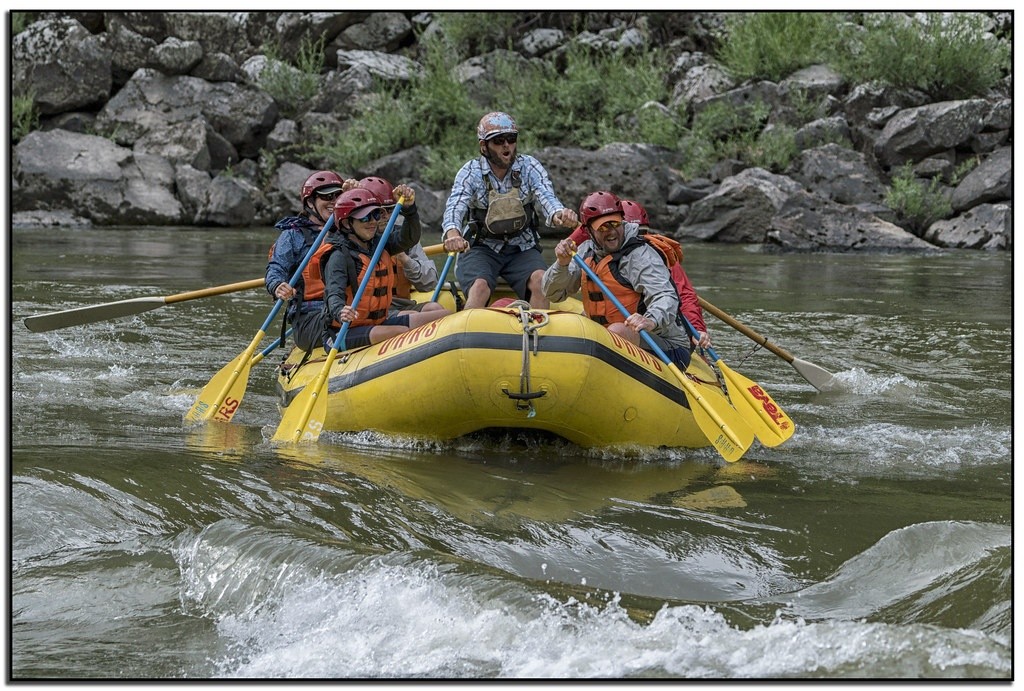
[271,194,405,442]
[24,243,449,332]
[567,240,755,462]
[697,295,832,390]
[433,250,457,301]
[185,210,334,422]
[681,315,796,448]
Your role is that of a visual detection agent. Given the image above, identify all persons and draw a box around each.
[540,191,691,372]
[359,176,444,316]
[442,111,578,312]
[620,201,713,349]
[266,169,363,352]
[321,188,456,355]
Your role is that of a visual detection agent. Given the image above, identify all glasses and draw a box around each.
[316,192,343,200]
[598,220,622,231]
[358,211,380,222]
[492,132,518,145]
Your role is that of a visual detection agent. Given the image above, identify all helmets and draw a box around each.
[620,200,649,225]
[301,170,344,209]
[477,111,518,138]
[579,190,625,224]
[359,176,396,204]
[333,189,382,229]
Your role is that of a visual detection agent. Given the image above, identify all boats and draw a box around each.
[280,281,717,457]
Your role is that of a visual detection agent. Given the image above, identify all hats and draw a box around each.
[589,212,622,230]
[317,187,343,194]
[350,205,387,218]
